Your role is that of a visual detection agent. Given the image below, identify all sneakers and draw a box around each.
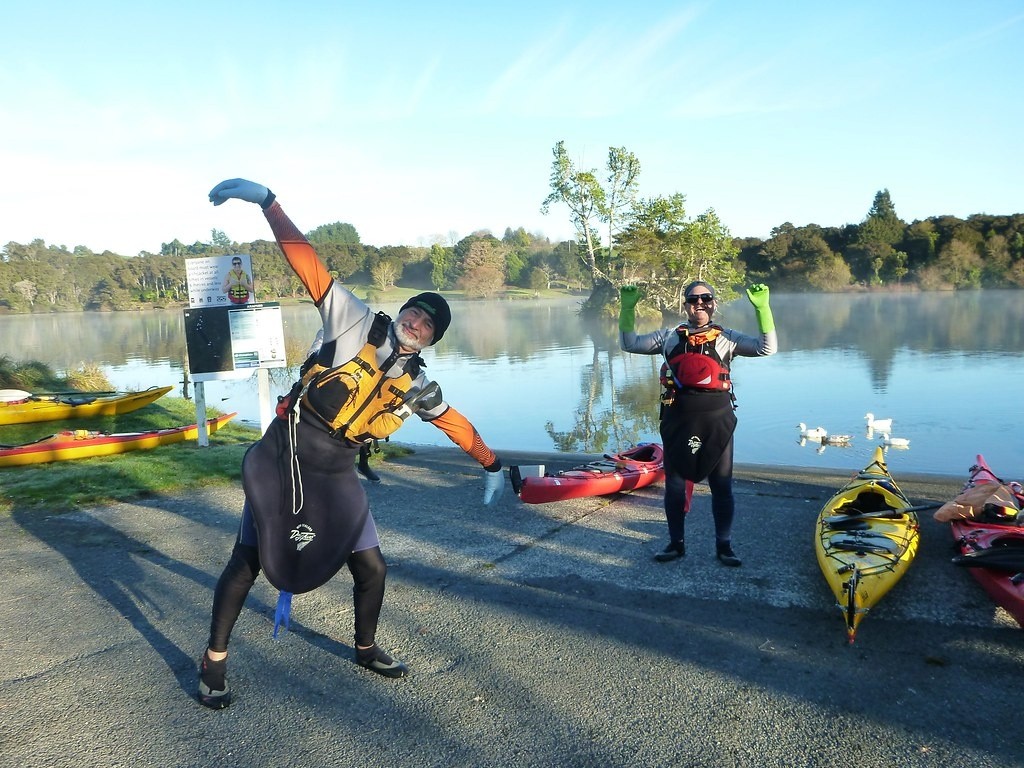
[354,642,410,677]
[197,647,231,709]
[716,537,741,566]
[655,539,685,561]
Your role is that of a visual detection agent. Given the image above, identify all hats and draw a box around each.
[400,292,451,345]
[684,281,714,298]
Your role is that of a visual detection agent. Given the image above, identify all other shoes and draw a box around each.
[357,462,381,483]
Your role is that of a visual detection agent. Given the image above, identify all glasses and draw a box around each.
[685,293,713,303]
[233,262,241,266]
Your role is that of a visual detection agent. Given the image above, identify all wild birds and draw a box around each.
[879,433,910,446]
[796,423,826,437]
[816,429,855,442]
[864,413,892,427]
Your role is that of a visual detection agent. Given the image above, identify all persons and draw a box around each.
[222,257,253,305]
[618,282,778,566]
[198,179,505,707]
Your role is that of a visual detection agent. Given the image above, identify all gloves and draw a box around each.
[618,285,642,332]
[482,456,505,508]
[746,283,775,333]
[208,178,276,209]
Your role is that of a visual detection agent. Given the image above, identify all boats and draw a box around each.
[814,445,921,645]
[510,442,665,504]
[0,409,240,468]
[949,454,1024,628]
[1,383,173,428]
